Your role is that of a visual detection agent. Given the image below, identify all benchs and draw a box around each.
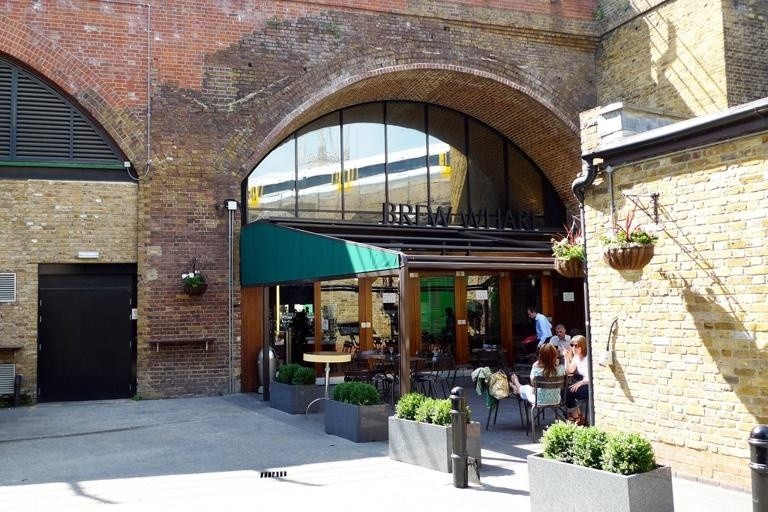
[0,363,21,406]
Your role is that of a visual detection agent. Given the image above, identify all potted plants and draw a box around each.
[270,363,318,414]
[526,420,673,511]
[325,381,389,442]
[388,392,481,473]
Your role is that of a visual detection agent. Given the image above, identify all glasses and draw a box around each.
[570,344,579,347]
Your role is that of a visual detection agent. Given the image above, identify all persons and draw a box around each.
[509,305,589,425]
[441,307,455,339]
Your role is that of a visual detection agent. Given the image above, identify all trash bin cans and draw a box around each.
[257,346,279,394]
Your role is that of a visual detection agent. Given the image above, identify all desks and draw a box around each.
[303,351,351,419]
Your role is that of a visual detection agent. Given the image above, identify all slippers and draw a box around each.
[510,376,520,395]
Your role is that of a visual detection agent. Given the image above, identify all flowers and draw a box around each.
[550,222,586,262]
[597,198,658,246]
[181,270,205,289]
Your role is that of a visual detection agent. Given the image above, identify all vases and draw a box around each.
[603,244,654,270]
[554,258,584,278]
[183,284,208,296]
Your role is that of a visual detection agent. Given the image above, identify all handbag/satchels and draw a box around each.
[489,370,509,400]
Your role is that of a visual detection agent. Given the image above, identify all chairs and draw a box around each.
[341,351,459,405]
[482,366,569,442]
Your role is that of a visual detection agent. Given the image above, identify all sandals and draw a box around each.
[568,413,581,424]
